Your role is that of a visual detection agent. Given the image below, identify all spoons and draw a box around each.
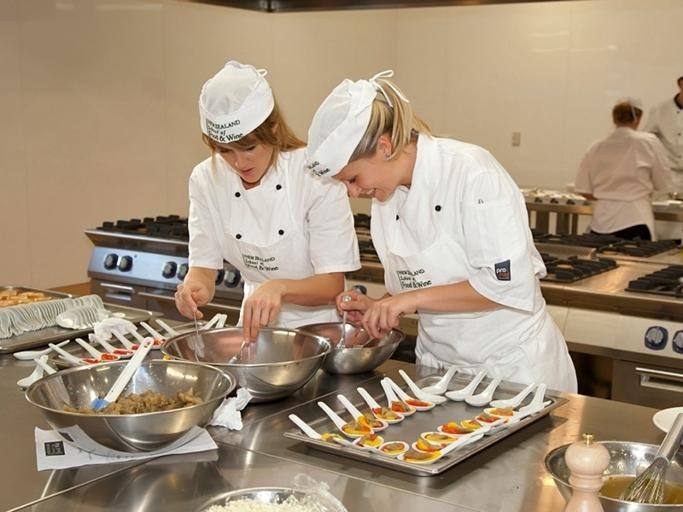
[14,311,225,388]
[0,294,103,340]
[289,371,556,469]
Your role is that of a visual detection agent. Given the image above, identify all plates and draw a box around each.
[196,484,345,512]
[653,404,683,432]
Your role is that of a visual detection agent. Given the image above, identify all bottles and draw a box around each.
[557,429,613,512]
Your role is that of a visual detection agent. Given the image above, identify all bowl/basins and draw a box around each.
[160,325,325,415]
[298,321,408,379]
[542,433,683,511]
[23,359,234,463]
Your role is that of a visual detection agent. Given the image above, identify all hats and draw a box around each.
[611,94,648,113]
[300,77,378,184]
[196,58,276,146]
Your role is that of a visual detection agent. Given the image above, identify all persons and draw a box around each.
[174,62,363,346]
[576,101,676,242]
[304,69,581,395]
[640,75,683,200]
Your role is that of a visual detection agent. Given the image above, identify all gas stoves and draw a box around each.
[526,225,682,265]
[81,216,250,303]
[348,240,682,363]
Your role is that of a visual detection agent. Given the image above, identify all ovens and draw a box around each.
[604,356,683,414]
[85,276,242,341]
